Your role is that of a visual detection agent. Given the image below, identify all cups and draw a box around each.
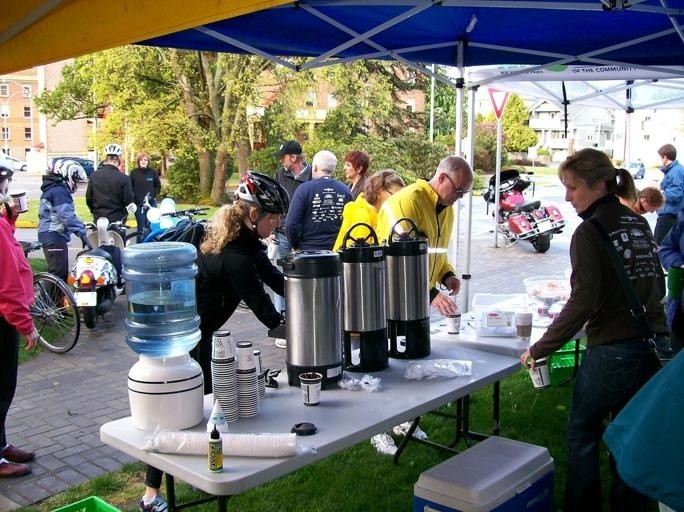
[153,430,208,459]
[296,371,322,407]
[516,310,532,344]
[9,190,29,215]
[444,312,461,336]
[526,358,550,389]
[211,330,266,423]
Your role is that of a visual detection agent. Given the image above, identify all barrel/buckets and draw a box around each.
[119,240,203,358]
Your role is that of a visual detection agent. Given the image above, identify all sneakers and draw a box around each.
[139,496,168,511]
[392,420,427,439]
[370,432,398,455]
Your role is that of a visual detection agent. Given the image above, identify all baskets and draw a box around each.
[547,340,587,386]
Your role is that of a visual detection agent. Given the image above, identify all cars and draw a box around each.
[621,159,646,182]
[47,156,95,183]
[0,155,29,173]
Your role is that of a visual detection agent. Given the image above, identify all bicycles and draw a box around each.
[6,240,79,356]
[123,191,287,308]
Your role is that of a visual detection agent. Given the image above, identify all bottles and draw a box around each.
[208,425,223,472]
[223,431,295,457]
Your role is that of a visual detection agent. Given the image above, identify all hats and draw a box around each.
[272,140,301,158]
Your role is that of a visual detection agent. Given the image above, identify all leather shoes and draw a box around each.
[0,443,34,462]
[0,458,30,477]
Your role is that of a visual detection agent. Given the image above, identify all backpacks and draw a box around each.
[142,219,207,251]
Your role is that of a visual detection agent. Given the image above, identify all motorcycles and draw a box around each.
[481,169,566,254]
[69,217,131,329]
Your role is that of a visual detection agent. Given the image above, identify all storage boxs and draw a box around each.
[472,292,530,337]
[413,435,556,512]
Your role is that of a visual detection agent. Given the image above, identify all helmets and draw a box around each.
[0,150,14,182]
[233,170,289,216]
[104,144,122,156]
[51,157,88,194]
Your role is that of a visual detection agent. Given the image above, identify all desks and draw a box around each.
[421,307,585,450]
[99,329,523,512]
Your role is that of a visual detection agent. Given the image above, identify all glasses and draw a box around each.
[446,175,470,194]
[383,188,393,196]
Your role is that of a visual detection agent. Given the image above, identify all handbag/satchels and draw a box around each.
[640,345,673,387]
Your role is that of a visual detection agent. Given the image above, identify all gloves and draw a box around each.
[79,233,93,251]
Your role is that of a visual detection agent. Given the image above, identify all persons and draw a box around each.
[136,170,290,512]
[37,153,162,318]
[617,143,684,361]
[521,147,667,512]
[266,140,369,347]
[0,153,41,478]
[366,153,475,456]
